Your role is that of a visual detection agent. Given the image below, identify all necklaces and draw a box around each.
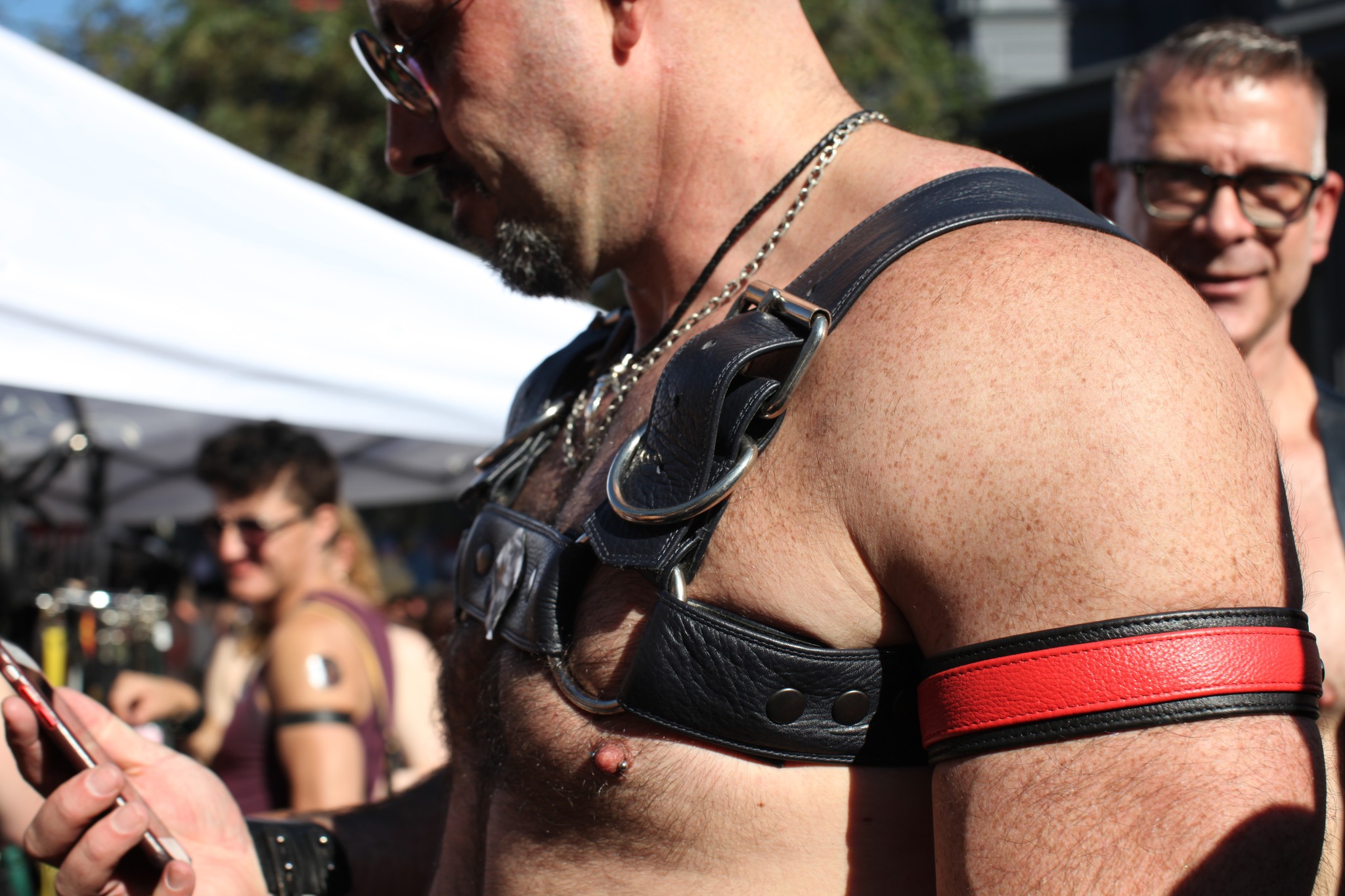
[552,107,889,512]
[583,105,876,425]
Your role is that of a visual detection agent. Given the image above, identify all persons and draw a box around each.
[1,0,1343,896]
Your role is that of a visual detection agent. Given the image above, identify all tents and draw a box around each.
[0,26,614,527]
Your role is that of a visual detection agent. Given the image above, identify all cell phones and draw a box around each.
[0,638,192,869]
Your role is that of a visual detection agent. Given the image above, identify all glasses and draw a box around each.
[349,0,467,115]
[202,514,312,549]
[1113,160,1330,231]
